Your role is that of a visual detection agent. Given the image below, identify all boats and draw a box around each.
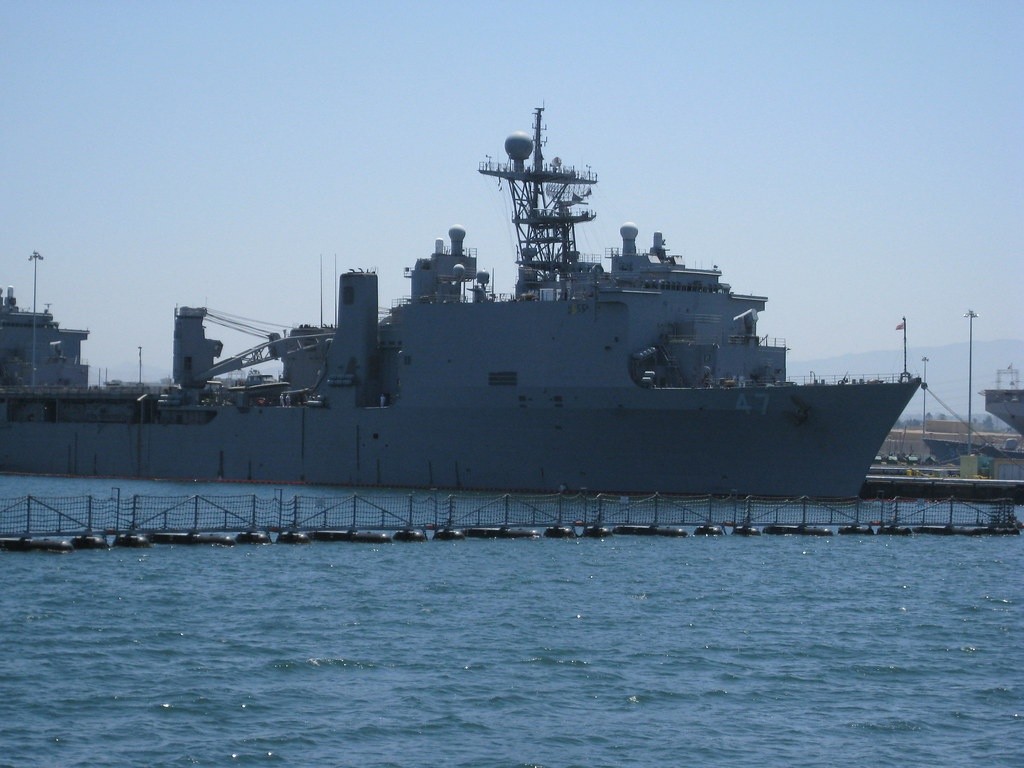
[923,438,1024,466]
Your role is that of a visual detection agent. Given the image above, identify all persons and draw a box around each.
[376,392,386,409]
[269,392,291,408]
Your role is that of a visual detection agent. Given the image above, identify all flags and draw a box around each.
[896,322,905,330]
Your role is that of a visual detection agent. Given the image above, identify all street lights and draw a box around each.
[28,249,44,394]
[138,345,143,385]
[960,309,979,479]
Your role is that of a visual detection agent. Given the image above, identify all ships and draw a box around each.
[984,360,1024,442]
[0,284,90,389]
[0,98,923,503]
